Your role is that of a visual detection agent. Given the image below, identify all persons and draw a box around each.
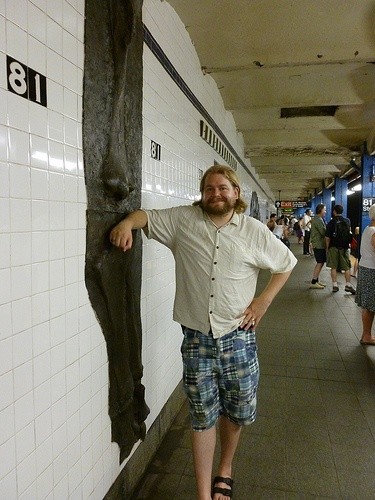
[303,208,312,256]
[340,226,361,277]
[308,204,327,290]
[354,203,375,345]
[109,165,299,499]
[324,204,356,295]
[267,212,316,255]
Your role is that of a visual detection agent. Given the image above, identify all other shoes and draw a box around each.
[333,286,339,292]
[318,281,326,286]
[310,282,324,289]
[345,285,356,294]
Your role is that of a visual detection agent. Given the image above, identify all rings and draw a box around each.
[251,317,255,325]
[111,239,115,242]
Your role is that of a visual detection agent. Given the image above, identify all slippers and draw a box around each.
[212,475,234,500]
[360,337,375,345]
